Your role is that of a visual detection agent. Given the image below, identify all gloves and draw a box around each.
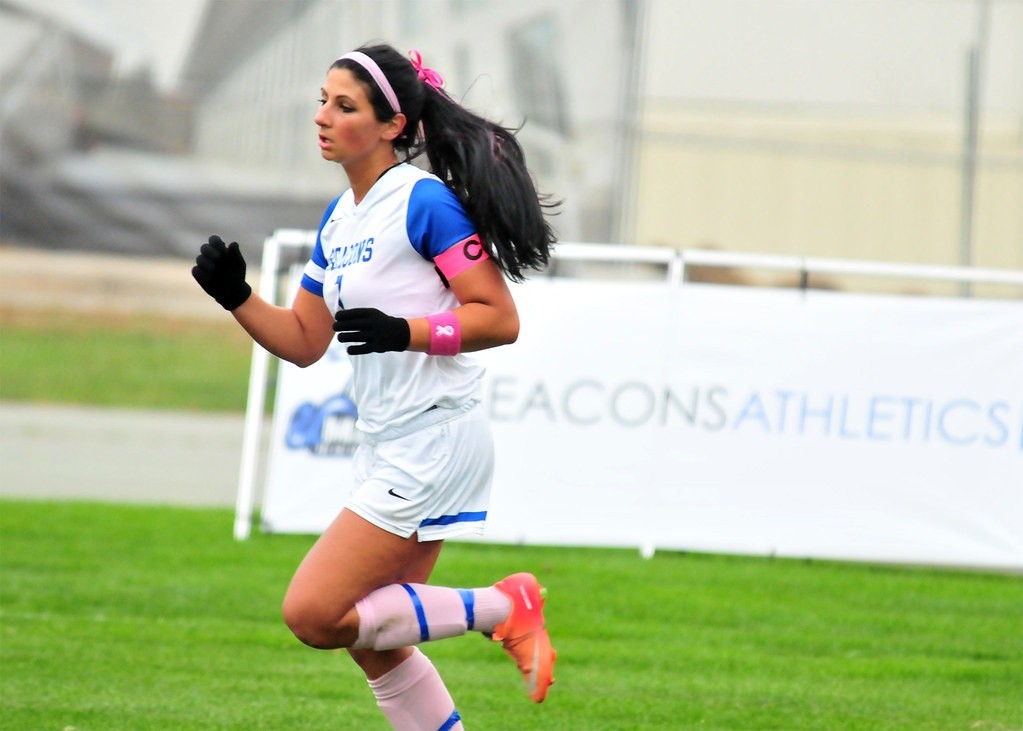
[331,308,411,356]
[192,234,253,312]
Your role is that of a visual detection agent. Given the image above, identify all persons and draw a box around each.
[192,44,563,730]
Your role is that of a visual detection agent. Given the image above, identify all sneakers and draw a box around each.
[484,572,556,704]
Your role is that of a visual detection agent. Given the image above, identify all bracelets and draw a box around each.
[425,310,460,356]
